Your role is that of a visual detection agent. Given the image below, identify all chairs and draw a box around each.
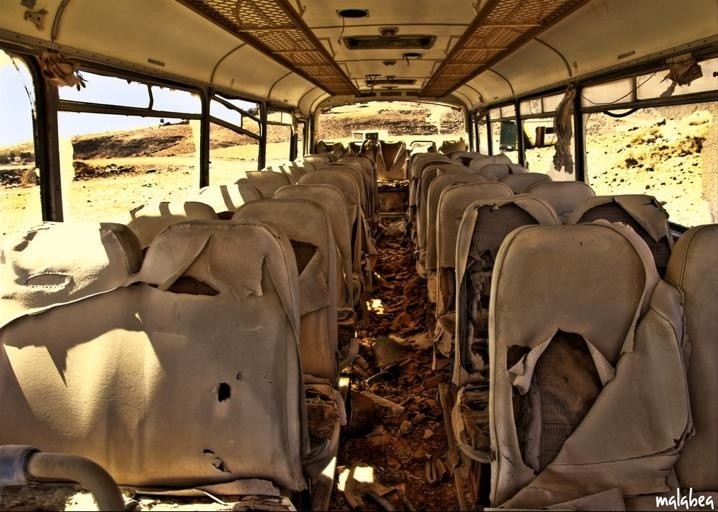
[407,147,717,508]
[310,139,468,209]
[0,146,391,511]
